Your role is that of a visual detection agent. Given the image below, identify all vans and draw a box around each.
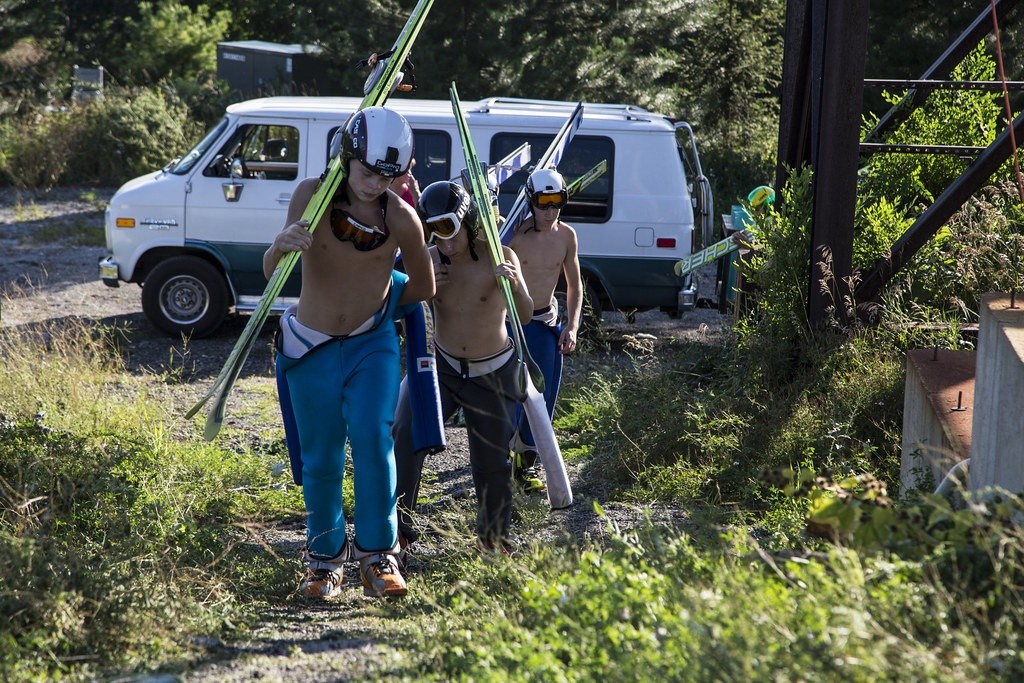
[99,96,715,340]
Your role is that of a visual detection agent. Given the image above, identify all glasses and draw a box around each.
[414,181,479,239]
[529,190,569,210]
[329,207,390,251]
[421,212,464,244]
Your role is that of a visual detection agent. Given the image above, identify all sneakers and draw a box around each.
[512,451,544,495]
[298,557,344,602]
[358,554,408,598]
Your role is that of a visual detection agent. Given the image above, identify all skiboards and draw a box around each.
[182,0,439,442]
[568,160,606,207]
[444,81,573,512]
[491,102,585,246]
[481,138,536,189]
[673,221,760,279]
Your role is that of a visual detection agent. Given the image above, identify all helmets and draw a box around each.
[524,169,567,207]
[341,106,415,178]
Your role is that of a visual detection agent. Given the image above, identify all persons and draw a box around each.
[262,107,436,600]
[392,180,534,571]
[506,169,583,492]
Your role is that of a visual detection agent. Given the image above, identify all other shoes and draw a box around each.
[477,535,517,553]
[398,531,436,556]
